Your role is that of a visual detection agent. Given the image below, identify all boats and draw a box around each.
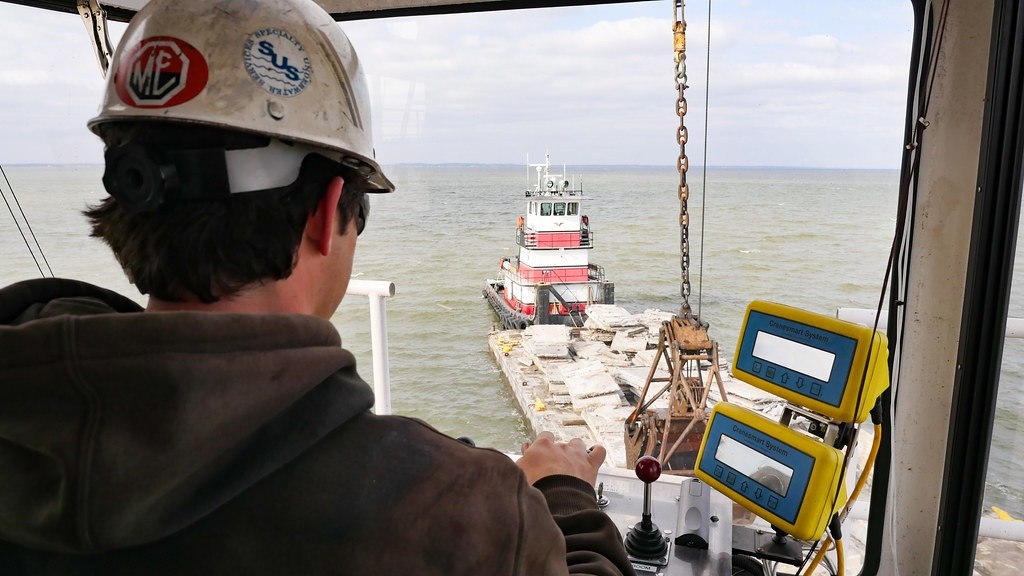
[482,143,616,331]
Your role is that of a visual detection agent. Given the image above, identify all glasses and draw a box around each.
[330,185,372,234]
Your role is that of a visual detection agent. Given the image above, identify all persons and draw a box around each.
[0,0,638,576]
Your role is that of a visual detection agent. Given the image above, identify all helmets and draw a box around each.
[88,1,400,195]
